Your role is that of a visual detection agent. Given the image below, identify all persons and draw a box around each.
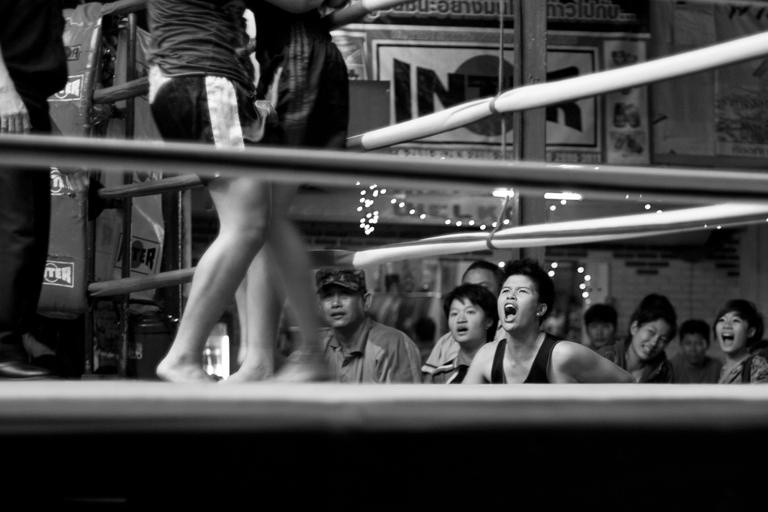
[310,267,424,384]
[596,292,767,384]
[144,0,271,385]
[0,0,90,378]
[423,260,635,383]
[218,1,352,387]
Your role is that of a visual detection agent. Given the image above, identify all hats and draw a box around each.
[311,264,372,297]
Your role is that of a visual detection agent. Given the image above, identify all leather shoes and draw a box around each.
[0,357,51,382]
